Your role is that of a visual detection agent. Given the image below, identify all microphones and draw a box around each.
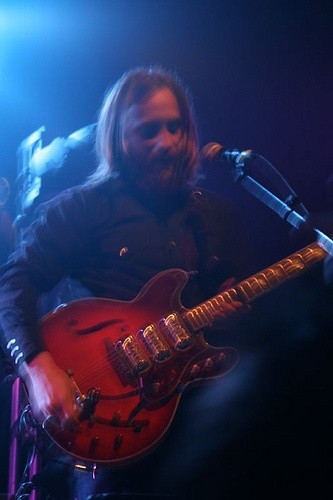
[201,142,255,164]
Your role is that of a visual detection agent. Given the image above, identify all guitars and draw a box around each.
[21,236,332,466]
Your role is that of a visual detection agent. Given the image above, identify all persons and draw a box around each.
[0,176,13,253]
[0,67,251,500]
